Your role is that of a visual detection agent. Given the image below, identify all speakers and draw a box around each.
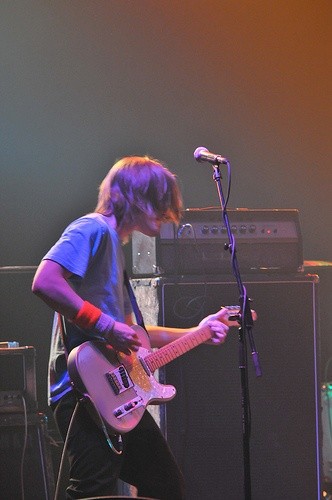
[1,413,59,500]
[0,266,54,411]
[117,272,326,500]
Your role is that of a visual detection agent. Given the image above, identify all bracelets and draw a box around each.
[95,311,116,335]
[72,300,102,330]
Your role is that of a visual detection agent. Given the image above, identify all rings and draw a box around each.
[127,342,131,348]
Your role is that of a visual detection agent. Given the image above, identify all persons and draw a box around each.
[32,157,230,500]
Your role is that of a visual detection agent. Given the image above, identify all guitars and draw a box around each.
[66,306,257,435]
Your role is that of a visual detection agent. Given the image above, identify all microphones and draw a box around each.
[193,146,228,165]
